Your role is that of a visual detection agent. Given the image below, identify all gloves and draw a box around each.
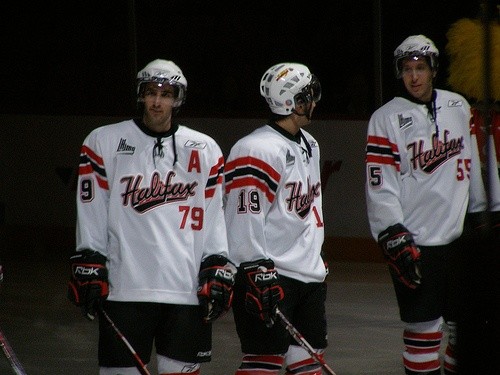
[197,254,238,324]
[321,250,330,280]
[67,249,109,321]
[377,223,423,289]
[240,259,285,328]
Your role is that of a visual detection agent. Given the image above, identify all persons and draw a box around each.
[226,62,329,375]
[364,0,500,375]
[67,59,238,375]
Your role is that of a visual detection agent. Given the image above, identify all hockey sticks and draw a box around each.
[101,308,151,375]
[0,332,27,375]
[276,308,337,375]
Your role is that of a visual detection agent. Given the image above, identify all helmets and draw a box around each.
[260,62,313,115]
[394,34,439,57]
[136,58,187,88]
